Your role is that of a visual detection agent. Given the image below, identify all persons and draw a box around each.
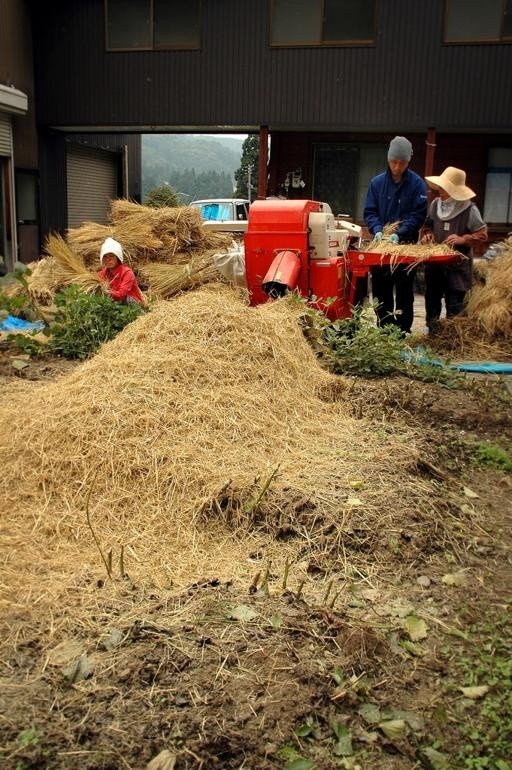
[419,165,488,333]
[362,134,431,341]
[85,235,149,319]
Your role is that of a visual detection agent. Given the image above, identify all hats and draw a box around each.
[423,165,476,201]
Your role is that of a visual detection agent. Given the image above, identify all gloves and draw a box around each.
[390,233,400,244]
[374,231,383,242]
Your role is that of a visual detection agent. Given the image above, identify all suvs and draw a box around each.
[187,198,251,223]
